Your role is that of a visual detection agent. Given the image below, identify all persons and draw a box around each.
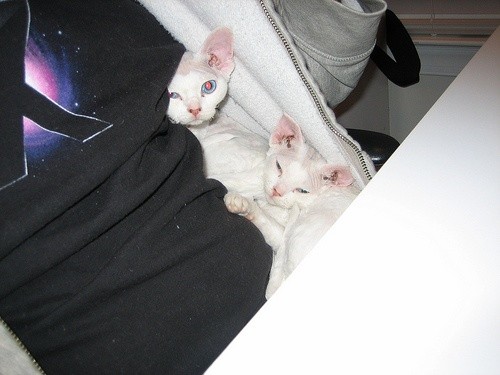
[0,0,397,375]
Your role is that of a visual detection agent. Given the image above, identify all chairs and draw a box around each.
[343,128,400,175]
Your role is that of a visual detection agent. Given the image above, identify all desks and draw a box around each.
[203,26,500,375]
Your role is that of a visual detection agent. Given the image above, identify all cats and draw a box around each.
[223,111,360,301]
[164,23,271,212]
